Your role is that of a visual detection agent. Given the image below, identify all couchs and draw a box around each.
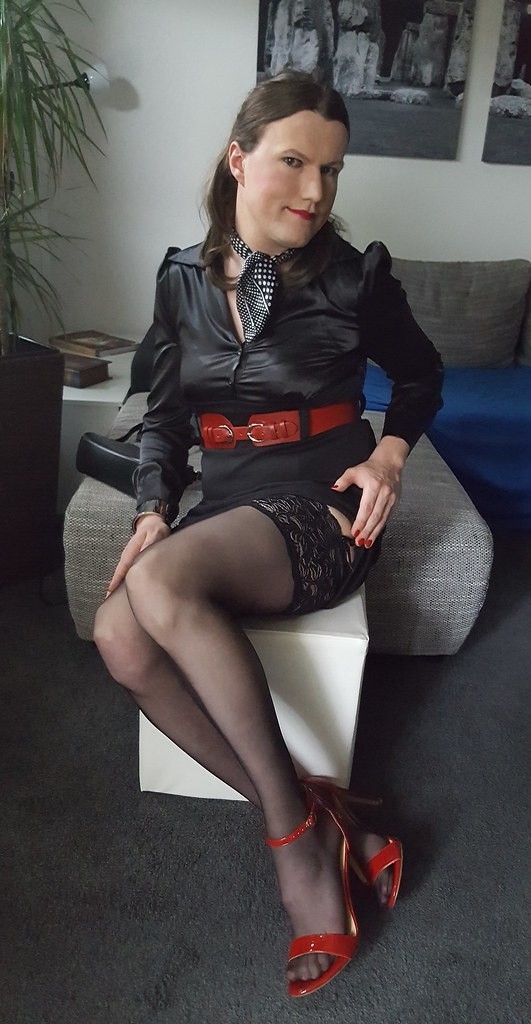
[64,259,531,654]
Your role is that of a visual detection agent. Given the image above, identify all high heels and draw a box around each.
[264,786,371,998]
[302,776,403,911]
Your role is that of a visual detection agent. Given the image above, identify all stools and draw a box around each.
[137,585,371,802]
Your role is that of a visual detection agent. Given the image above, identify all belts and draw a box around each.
[196,397,362,452]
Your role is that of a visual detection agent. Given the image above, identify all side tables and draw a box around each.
[59,347,139,526]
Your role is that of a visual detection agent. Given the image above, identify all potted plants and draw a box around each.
[0,4,99,587]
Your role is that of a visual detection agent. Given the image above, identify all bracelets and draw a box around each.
[132,500,180,534]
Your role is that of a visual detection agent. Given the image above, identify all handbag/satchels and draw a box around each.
[74,423,197,498]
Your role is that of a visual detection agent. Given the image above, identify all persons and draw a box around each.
[91,71,446,1000]
